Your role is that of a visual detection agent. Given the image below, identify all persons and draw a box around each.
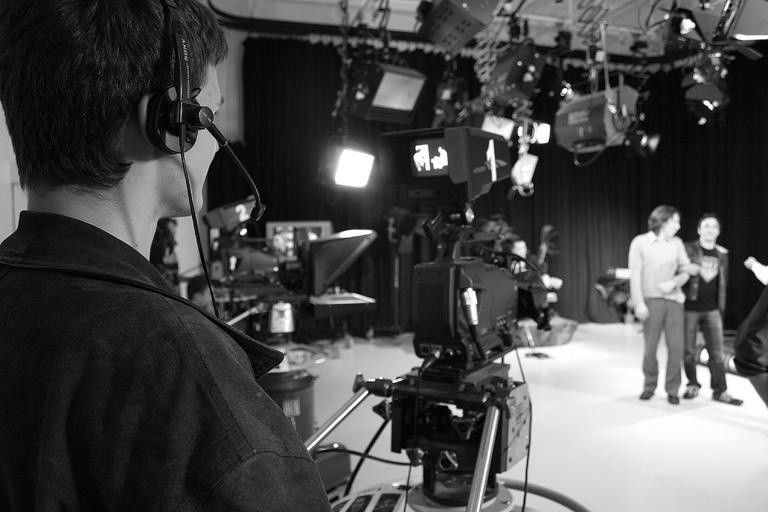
[677,212,744,408]
[1,1,334,511]
[509,237,563,332]
[627,204,691,405]
[186,275,214,310]
[158,217,180,295]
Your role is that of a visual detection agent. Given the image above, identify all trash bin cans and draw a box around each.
[258,368,315,444]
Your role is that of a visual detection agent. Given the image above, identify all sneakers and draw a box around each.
[640,390,653,399]
[669,395,679,404]
[714,391,743,405]
[685,385,700,399]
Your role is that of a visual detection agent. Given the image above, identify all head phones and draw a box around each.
[147,0,199,155]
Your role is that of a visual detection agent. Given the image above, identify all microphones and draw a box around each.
[196,106,267,223]
